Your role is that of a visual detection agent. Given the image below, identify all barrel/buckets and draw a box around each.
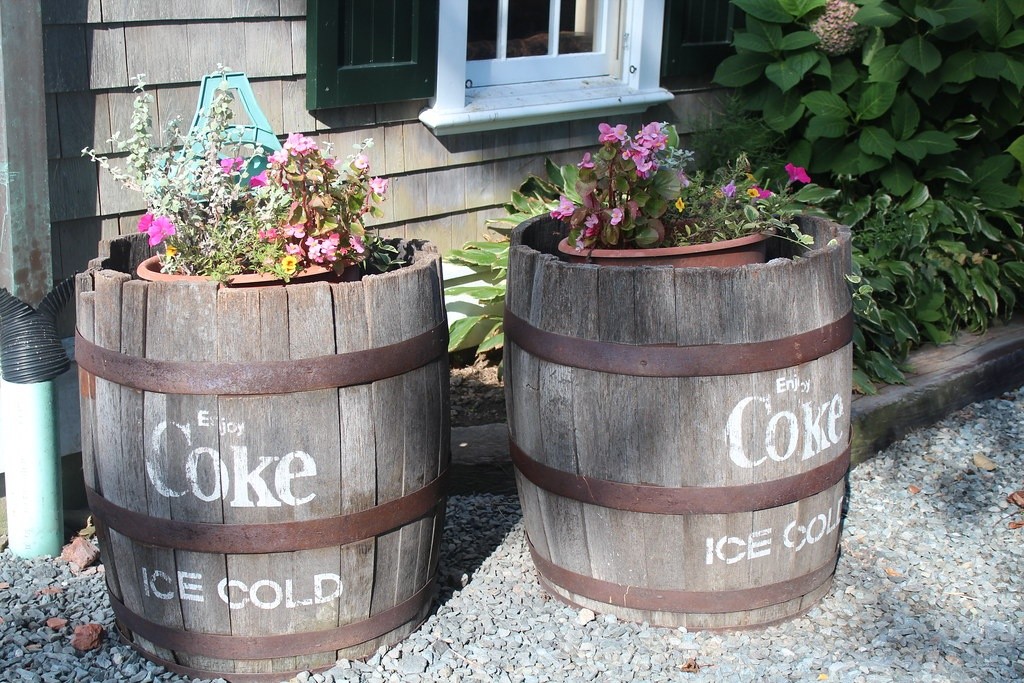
[511,210,853,628]
[73,232,450,683]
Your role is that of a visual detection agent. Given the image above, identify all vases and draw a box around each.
[557,226,777,265]
[137,252,370,288]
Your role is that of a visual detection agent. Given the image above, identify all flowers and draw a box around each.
[549,121,842,264]
[80,70,389,282]
